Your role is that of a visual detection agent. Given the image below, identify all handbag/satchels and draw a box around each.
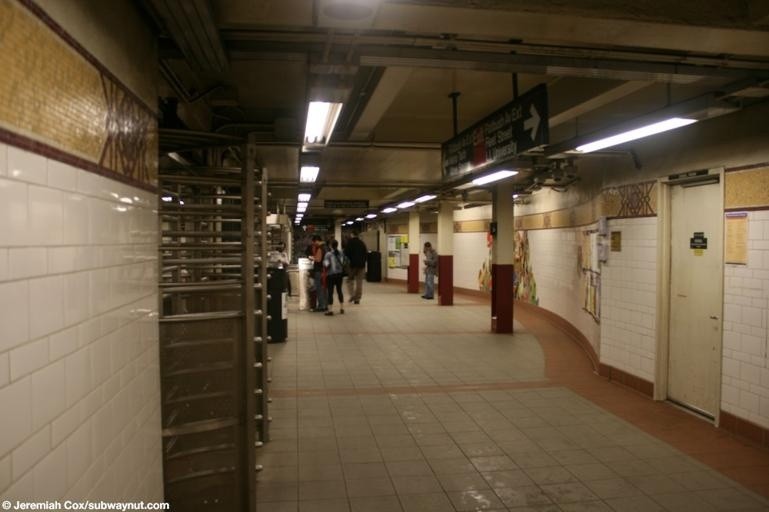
[344,266,349,275]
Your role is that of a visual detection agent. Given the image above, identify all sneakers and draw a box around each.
[420,296,434,299]
[354,300,359,303]
[349,296,354,301]
[340,309,344,313]
[324,312,334,316]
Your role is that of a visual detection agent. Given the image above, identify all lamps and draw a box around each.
[380,193,437,214]
[297,100,345,202]
[472,169,519,187]
[575,117,699,153]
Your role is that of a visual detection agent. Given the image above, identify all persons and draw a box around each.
[311,236,329,312]
[420,242,438,298]
[323,240,346,315]
[305,237,318,309]
[342,229,368,305]
